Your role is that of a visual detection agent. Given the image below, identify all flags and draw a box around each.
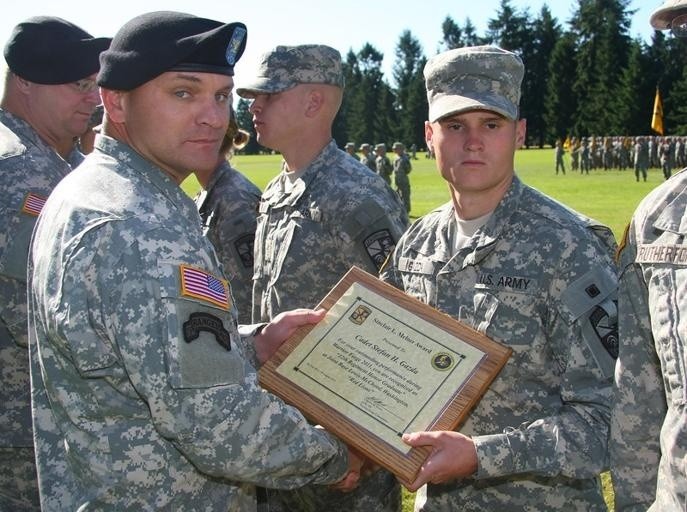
[649,92,664,136]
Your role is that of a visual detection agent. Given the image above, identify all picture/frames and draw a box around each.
[254,263,514,484]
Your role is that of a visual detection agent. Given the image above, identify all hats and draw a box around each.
[392,142,404,150]
[7,19,111,86]
[423,42,525,124]
[373,143,386,152]
[344,142,355,149]
[235,43,346,100]
[98,9,248,92]
[359,143,370,151]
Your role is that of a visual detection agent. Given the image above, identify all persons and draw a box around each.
[236,40,410,511]
[0,14,112,512]
[25,10,367,512]
[54,104,104,169]
[191,100,265,327]
[550,135,685,182]
[610,0,685,511]
[333,45,622,512]
[342,139,437,217]
